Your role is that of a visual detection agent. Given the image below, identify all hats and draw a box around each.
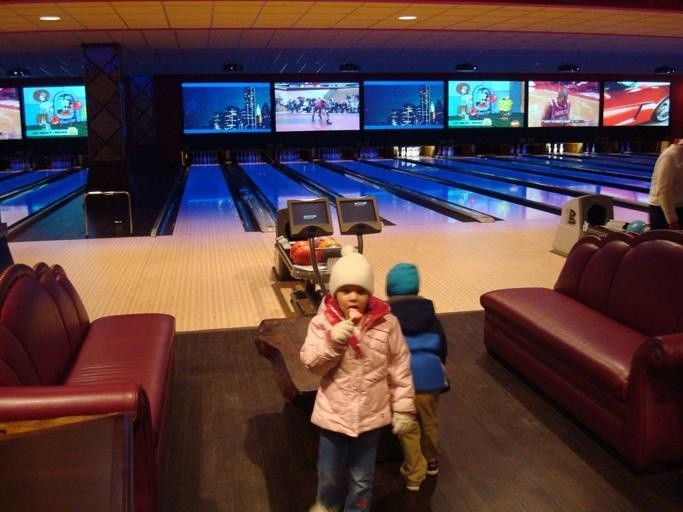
[328,245,374,299]
[386,263,419,297]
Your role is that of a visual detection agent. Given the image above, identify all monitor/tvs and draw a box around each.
[288,197,333,238]
[447,80,526,128]
[274,82,360,132]
[180,82,272,134]
[22,84,89,138]
[363,80,446,130]
[527,80,600,128]
[336,197,382,235]
[602,80,672,127]
[0,88,23,140]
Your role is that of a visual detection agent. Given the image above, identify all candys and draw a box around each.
[348,312,355,321]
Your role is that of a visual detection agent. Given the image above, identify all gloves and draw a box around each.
[331,319,354,345]
[392,412,413,436]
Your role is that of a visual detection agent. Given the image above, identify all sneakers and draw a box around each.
[427,459,439,475]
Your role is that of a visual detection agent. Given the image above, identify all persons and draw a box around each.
[541,90,571,128]
[648,134,683,230]
[299,255,417,511]
[384,263,450,492]
[276,94,362,126]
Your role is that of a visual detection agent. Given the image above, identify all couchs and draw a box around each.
[0,261,176,511]
[479,229,683,472]
